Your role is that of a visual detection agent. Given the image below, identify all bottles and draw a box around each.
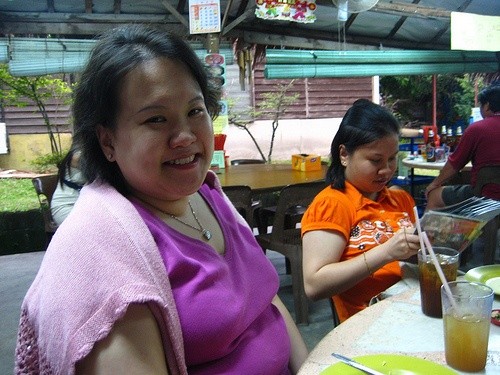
[439,125,462,153]
[426,128,436,162]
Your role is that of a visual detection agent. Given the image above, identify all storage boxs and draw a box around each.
[291,154,321,172]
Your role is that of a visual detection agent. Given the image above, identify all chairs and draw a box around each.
[456,167,500,266]
[32,175,58,233]
[222,181,329,326]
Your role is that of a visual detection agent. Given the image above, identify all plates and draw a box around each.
[464,264,500,296]
[317,354,461,375]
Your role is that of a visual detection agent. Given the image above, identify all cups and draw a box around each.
[441,281,494,372]
[417,247,459,318]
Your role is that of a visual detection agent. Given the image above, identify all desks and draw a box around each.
[209,164,329,275]
[402,154,473,198]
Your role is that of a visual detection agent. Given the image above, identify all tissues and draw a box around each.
[292,152,321,171]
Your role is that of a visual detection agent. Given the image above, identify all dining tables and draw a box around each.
[295,277,500,374]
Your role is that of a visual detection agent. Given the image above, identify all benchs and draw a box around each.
[388,136,434,203]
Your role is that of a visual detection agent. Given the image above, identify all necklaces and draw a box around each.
[156,200,213,241]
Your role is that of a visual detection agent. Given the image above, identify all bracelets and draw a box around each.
[362,251,374,275]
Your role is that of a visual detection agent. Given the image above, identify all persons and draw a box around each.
[50,148,89,229]
[425,84,500,214]
[15,20,311,375]
[301,99,425,325]
